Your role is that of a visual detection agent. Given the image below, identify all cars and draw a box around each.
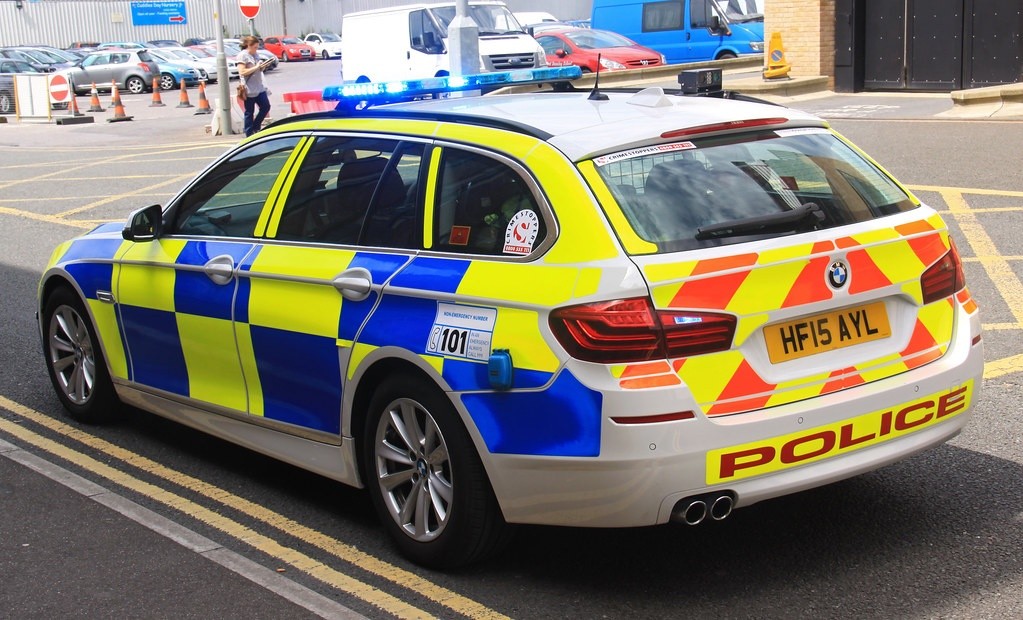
[35,66,985,569]
[495,13,666,74]
[304,33,342,59]
[262,35,316,62]
[0,37,278,115]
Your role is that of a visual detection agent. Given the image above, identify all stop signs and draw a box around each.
[47,73,72,104]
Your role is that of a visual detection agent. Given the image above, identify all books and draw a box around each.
[259,57,275,66]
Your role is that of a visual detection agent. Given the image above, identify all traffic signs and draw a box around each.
[130,1,188,26]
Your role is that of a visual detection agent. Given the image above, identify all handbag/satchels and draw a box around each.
[236,85,246,101]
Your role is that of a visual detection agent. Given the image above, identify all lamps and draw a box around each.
[16,0,22,10]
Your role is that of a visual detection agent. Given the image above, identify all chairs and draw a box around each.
[319,156,406,245]
[447,170,520,244]
[640,159,709,202]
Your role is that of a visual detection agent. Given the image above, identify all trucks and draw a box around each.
[341,2,548,86]
[591,0,765,63]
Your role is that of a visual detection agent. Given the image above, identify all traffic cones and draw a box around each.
[87,82,106,113]
[106,88,134,121]
[196,86,211,114]
[66,85,85,117]
[201,81,213,111]
[110,79,124,109]
[147,77,166,107]
[176,77,194,109]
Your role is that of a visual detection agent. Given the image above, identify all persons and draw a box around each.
[236,36,272,138]
